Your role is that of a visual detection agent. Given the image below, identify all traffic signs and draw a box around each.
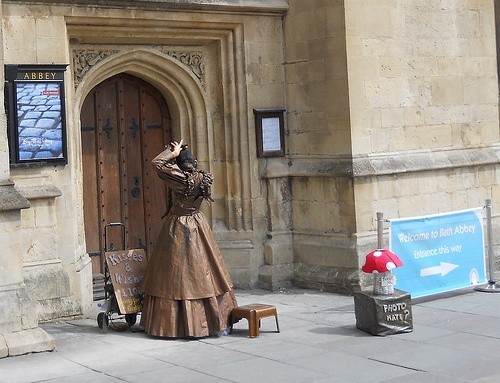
[388,208,487,300]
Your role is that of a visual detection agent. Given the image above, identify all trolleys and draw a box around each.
[98,222,140,329]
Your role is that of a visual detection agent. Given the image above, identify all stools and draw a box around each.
[230,303,281,338]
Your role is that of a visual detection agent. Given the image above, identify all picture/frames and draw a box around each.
[253,107,286,158]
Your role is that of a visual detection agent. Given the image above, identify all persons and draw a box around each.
[140,139,244,341]
